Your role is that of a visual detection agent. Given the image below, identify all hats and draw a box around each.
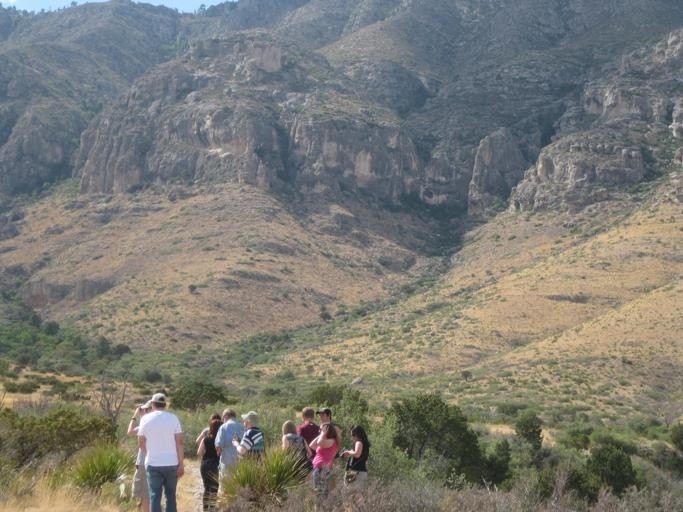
[316,408,332,415]
[152,392,166,403]
[141,400,152,409]
[241,411,260,428]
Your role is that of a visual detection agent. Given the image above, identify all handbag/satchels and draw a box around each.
[344,471,358,483]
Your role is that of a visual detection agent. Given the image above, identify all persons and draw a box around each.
[127,391,186,512]
[195,408,266,512]
[281,406,371,512]
